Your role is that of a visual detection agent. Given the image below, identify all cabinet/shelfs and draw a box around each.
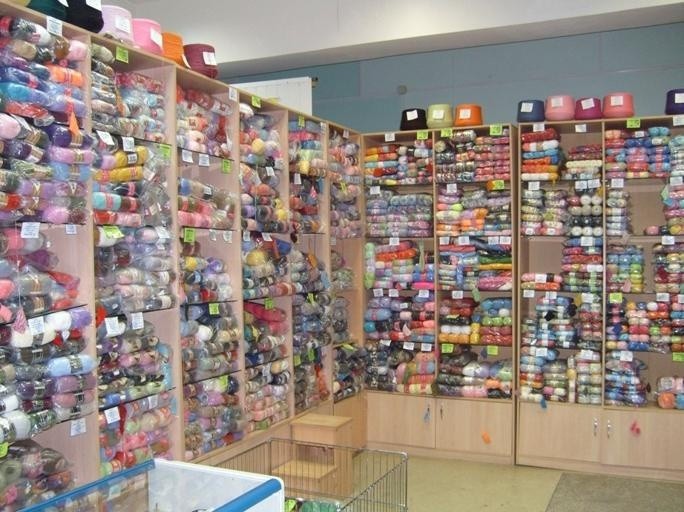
[519,122,605,473]
[325,119,366,455]
[269,414,355,502]
[0,1,97,512]
[90,33,182,506]
[604,116,683,478]
[177,63,239,469]
[239,89,290,453]
[289,108,327,417]
[433,123,517,464]
[360,128,434,456]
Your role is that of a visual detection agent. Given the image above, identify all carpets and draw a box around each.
[540,467,683,512]
